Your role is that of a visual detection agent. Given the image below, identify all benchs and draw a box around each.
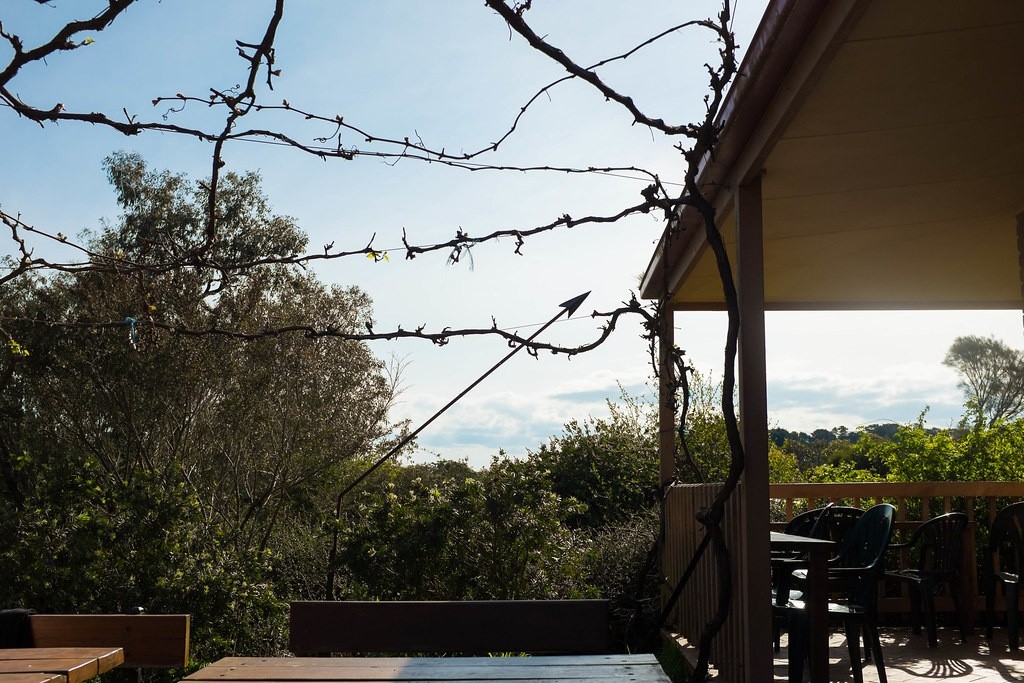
[0,614,190,667]
[289,599,610,656]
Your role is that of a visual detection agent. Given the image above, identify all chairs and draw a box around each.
[884,511,969,647]
[983,499,1024,650]
[775,507,876,661]
[791,503,898,683]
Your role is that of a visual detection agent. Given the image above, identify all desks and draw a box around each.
[771,531,837,683]
[0,646,124,683]
[177,652,673,683]
